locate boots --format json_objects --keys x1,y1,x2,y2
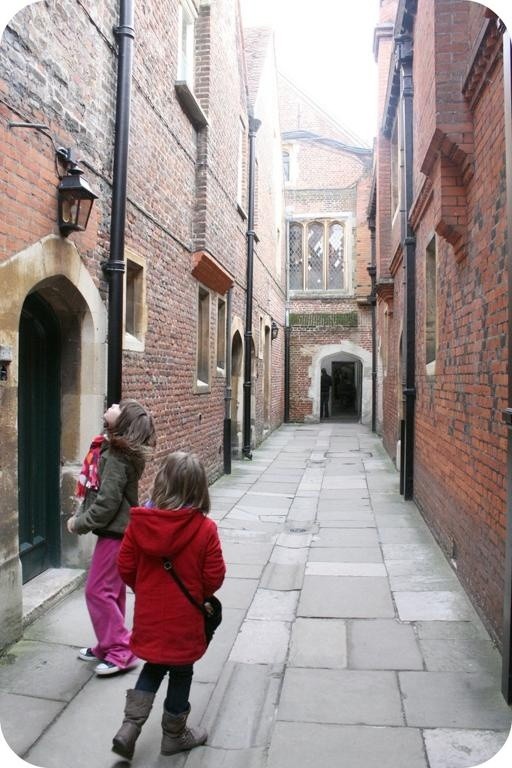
[
  {"x1": 160, "y1": 699, "x2": 208, "y2": 756},
  {"x1": 111, "y1": 688, "x2": 155, "y2": 761}
]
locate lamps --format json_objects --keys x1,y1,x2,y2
[{"x1": 8, "y1": 122, "x2": 98, "y2": 237}]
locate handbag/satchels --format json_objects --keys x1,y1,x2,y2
[{"x1": 203, "y1": 594, "x2": 222, "y2": 647}]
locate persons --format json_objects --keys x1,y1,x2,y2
[
  {"x1": 106, "y1": 446, "x2": 227, "y2": 759},
  {"x1": 67, "y1": 396, "x2": 160, "y2": 679},
  {"x1": 320, "y1": 368, "x2": 333, "y2": 420}
]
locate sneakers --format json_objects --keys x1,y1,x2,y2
[
  {"x1": 79, "y1": 646, "x2": 101, "y2": 662},
  {"x1": 94, "y1": 654, "x2": 140, "y2": 675}
]
